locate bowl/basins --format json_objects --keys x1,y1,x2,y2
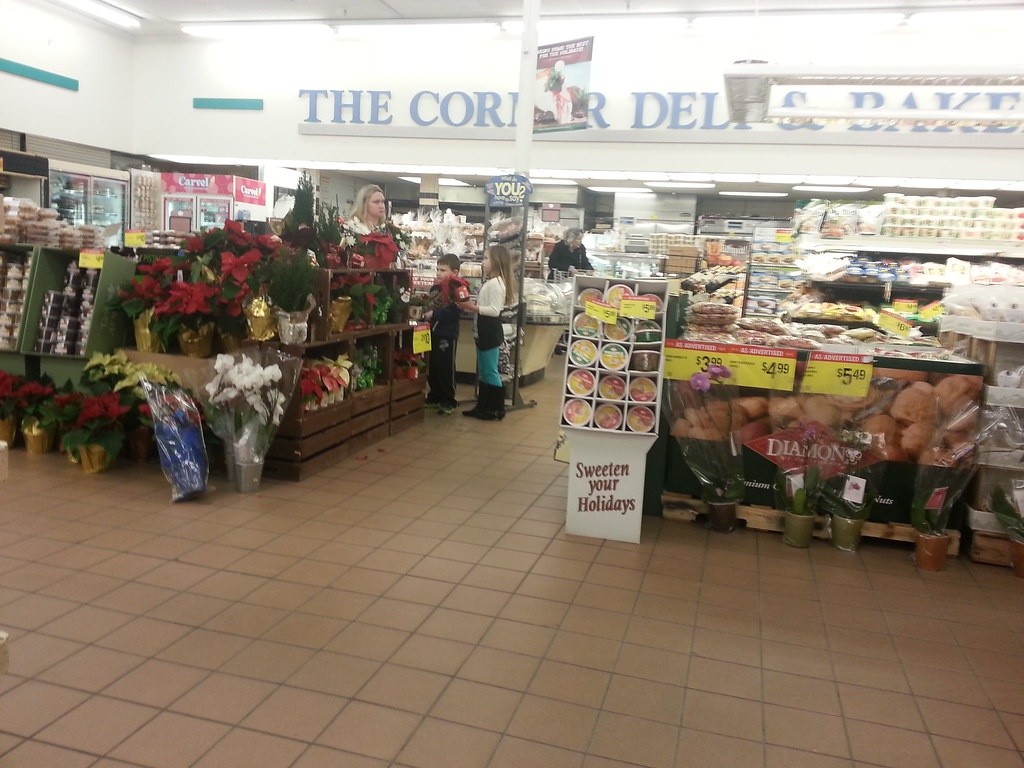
[{"x1": 882, "y1": 192, "x2": 1024, "y2": 241}]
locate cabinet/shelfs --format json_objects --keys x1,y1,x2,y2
[
  {"x1": 788, "y1": 233, "x2": 1024, "y2": 337},
  {"x1": 741, "y1": 252, "x2": 800, "y2": 317}
]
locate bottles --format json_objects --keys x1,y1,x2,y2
[{"x1": 680, "y1": 264, "x2": 747, "y2": 305}]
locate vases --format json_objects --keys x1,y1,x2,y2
[
  {"x1": 0, "y1": 416, "x2": 17, "y2": 449},
  {"x1": 235, "y1": 461, "x2": 264, "y2": 492},
  {"x1": 78, "y1": 444, "x2": 112, "y2": 473},
  {"x1": 274, "y1": 294, "x2": 316, "y2": 344},
  {"x1": 331, "y1": 297, "x2": 352, "y2": 332},
  {"x1": 832, "y1": 514, "x2": 863, "y2": 551},
  {"x1": 405, "y1": 367, "x2": 418, "y2": 378},
  {"x1": 244, "y1": 295, "x2": 277, "y2": 342},
  {"x1": 306, "y1": 385, "x2": 343, "y2": 411},
  {"x1": 782, "y1": 508, "x2": 817, "y2": 547},
  {"x1": 22, "y1": 421, "x2": 55, "y2": 453},
  {"x1": 133, "y1": 307, "x2": 166, "y2": 353},
  {"x1": 127, "y1": 428, "x2": 151, "y2": 456},
  {"x1": 707, "y1": 501, "x2": 736, "y2": 533},
  {"x1": 177, "y1": 321, "x2": 215, "y2": 358}
]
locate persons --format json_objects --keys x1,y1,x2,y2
[
  {"x1": 422, "y1": 254, "x2": 461, "y2": 416},
  {"x1": 349, "y1": 184, "x2": 385, "y2": 227},
  {"x1": 547, "y1": 227, "x2": 594, "y2": 355},
  {"x1": 455, "y1": 245, "x2": 516, "y2": 421}
]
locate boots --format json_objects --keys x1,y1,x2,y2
[
  {"x1": 462, "y1": 382, "x2": 486, "y2": 416},
  {"x1": 476, "y1": 384, "x2": 507, "y2": 419}
]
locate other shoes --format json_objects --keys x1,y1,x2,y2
[
  {"x1": 437, "y1": 402, "x2": 457, "y2": 415},
  {"x1": 424, "y1": 399, "x2": 440, "y2": 408}
]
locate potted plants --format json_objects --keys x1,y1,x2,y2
[
  {"x1": 992, "y1": 485, "x2": 1024, "y2": 577},
  {"x1": 910, "y1": 467, "x2": 959, "y2": 571}
]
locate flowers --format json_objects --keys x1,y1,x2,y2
[
  {"x1": 781, "y1": 420, "x2": 877, "y2": 520},
  {"x1": 0, "y1": 350, "x2": 204, "y2": 473},
  {"x1": 301, "y1": 200, "x2": 426, "y2": 406},
  {"x1": 689, "y1": 364, "x2": 745, "y2": 503},
  {"x1": 206, "y1": 353, "x2": 285, "y2": 460},
  {"x1": 108, "y1": 220, "x2": 298, "y2": 354}
]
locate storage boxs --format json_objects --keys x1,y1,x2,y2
[{"x1": 663, "y1": 243, "x2": 699, "y2": 275}]
